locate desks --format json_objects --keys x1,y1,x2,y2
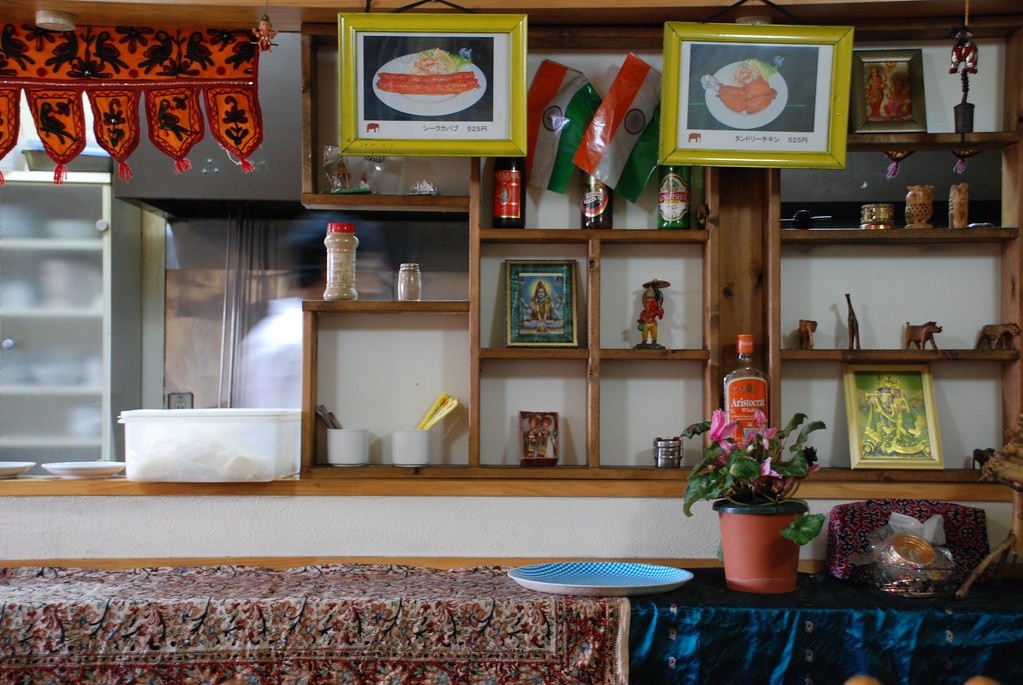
[{"x1": 1, "y1": 567, "x2": 1023, "y2": 685}]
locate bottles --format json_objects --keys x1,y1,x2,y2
[
  {"x1": 398, "y1": 263, "x2": 421, "y2": 301},
  {"x1": 581, "y1": 171, "x2": 612, "y2": 230},
  {"x1": 721, "y1": 333, "x2": 768, "y2": 443},
  {"x1": 323, "y1": 223, "x2": 360, "y2": 300},
  {"x1": 492, "y1": 156, "x2": 526, "y2": 229},
  {"x1": 658, "y1": 164, "x2": 691, "y2": 230}
]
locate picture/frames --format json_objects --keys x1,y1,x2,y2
[
  {"x1": 504, "y1": 259, "x2": 576, "y2": 347},
  {"x1": 519, "y1": 410, "x2": 560, "y2": 463},
  {"x1": 843, "y1": 362, "x2": 944, "y2": 469},
  {"x1": 656, "y1": 21, "x2": 855, "y2": 170},
  {"x1": 852, "y1": 48, "x2": 928, "y2": 132},
  {"x1": 337, "y1": 12, "x2": 528, "y2": 158}
]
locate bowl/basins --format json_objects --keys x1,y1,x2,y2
[{"x1": 873, "y1": 562, "x2": 954, "y2": 597}]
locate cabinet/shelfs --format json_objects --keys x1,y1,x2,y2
[
  {"x1": 0, "y1": 170, "x2": 141, "y2": 466},
  {"x1": 301, "y1": 0, "x2": 1023, "y2": 481}
]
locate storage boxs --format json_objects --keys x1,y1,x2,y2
[{"x1": 120, "y1": 407, "x2": 301, "y2": 482}]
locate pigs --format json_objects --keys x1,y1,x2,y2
[{"x1": 905, "y1": 322, "x2": 942, "y2": 351}]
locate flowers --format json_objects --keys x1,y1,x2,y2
[{"x1": 681, "y1": 400, "x2": 827, "y2": 514}]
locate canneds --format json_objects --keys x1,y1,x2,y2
[
  {"x1": 324, "y1": 223, "x2": 359, "y2": 300},
  {"x1": 396, "y1": 264, "x2": 422, "y2": 302}
]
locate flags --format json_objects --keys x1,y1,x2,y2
[
  {"x1": 525, "y1": 59, "x2": 604, "y2": 193},
  {"x1": 571, "y1": 52, "x2": 665, "y2": 204}
]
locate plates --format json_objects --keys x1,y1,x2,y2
[
  {"x1": 507, "y1": 562, "x2": 694, "y2": 598},
  {"x1": 372, "y1": 53, "x2": 486, "y2": 116},
  {"x1": 41, "y1": 461, "x2": 125, "y2": 480},
  {"x1": 0, "y1": 461, "x2": 36, "y2": 479},
  {"x1": 705, "y1": 61, "x2": 788, "y2": 129}
]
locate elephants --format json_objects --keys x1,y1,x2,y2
[{"x1": 798, "y1": 319, "x2": 817, "y2": 351}]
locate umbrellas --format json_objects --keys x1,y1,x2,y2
[{"x1": 642, "y1": 279, "x2": 670, "y2": 308}]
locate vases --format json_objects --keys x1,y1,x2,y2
[{"x1": 714, "y1": 503, "x2": 810, "y2": 593}]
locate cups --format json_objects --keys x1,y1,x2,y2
[
  {"x1": 326, "y1": 426, "x2": 369, "y2": 467},
  {"x1": 391, "y1": 428, "x2": 432, "y2": 468}
]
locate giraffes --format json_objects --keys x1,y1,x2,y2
[{"x1": 845, "y1": 294, "x2": 861, "y2": 349}]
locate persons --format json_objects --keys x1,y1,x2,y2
[
  {"x1": 252, "y1": 20, "x2": 277, "y2": 53},
  {"x1": 522, "y1": 416, "x2": 552, "y2": 458},
  {"x1": 637, "y1": 286, "x2": 664, "y2": 344},
  {"x1": 243, "y1": 215, "x2": 329, "y2": 410}
]
